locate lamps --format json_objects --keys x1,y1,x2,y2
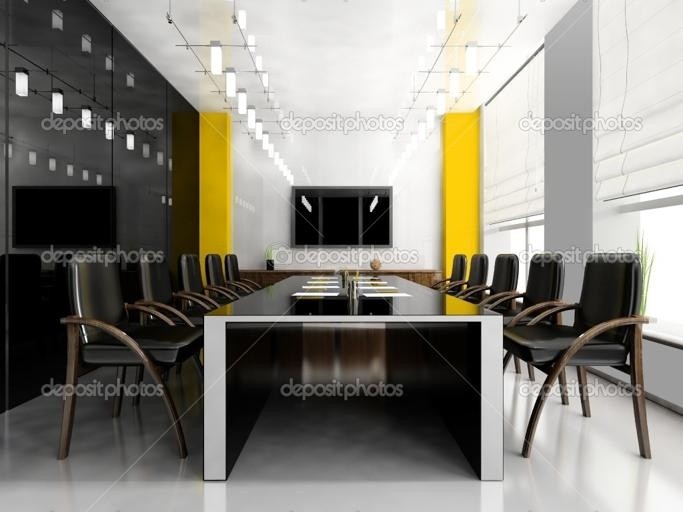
[
  {"x1": 1, "y1": 0, "x2": 173, "y2": 207},
  {"x1": 230, "y1": 2, "x2": 270, "y2": 89},
  {"x1": 164, "y1": 0, "x2": 295, "y2": 187},
  {"x1": 409, "y1": 6, "x2": 476, "y2": 150}
]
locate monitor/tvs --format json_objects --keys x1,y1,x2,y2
[
  {"x1": 12, "y1": 186, "x2": 117, "y2": 247},
  {"x1": 291, "y1": 185, "x2": 393, "y2": 247}
]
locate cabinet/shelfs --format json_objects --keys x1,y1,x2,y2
[{"x1": 240, "y1": 268, "x2": 443, "y2": 289}]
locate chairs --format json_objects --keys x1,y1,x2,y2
[
  {"x1": 429, "y1": 253, "x2": 653, "y2": 460},
  {"x1": 57, "y1": 254, "x2": 263, "y2": 460}
]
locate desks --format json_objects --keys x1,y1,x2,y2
[{"x1": 203, "y1": 274, "x2": 504, "y2": 482}]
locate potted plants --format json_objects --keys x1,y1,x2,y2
[{"x1": 265, "y1": 246, "x2": 276, "y2": 270}]
